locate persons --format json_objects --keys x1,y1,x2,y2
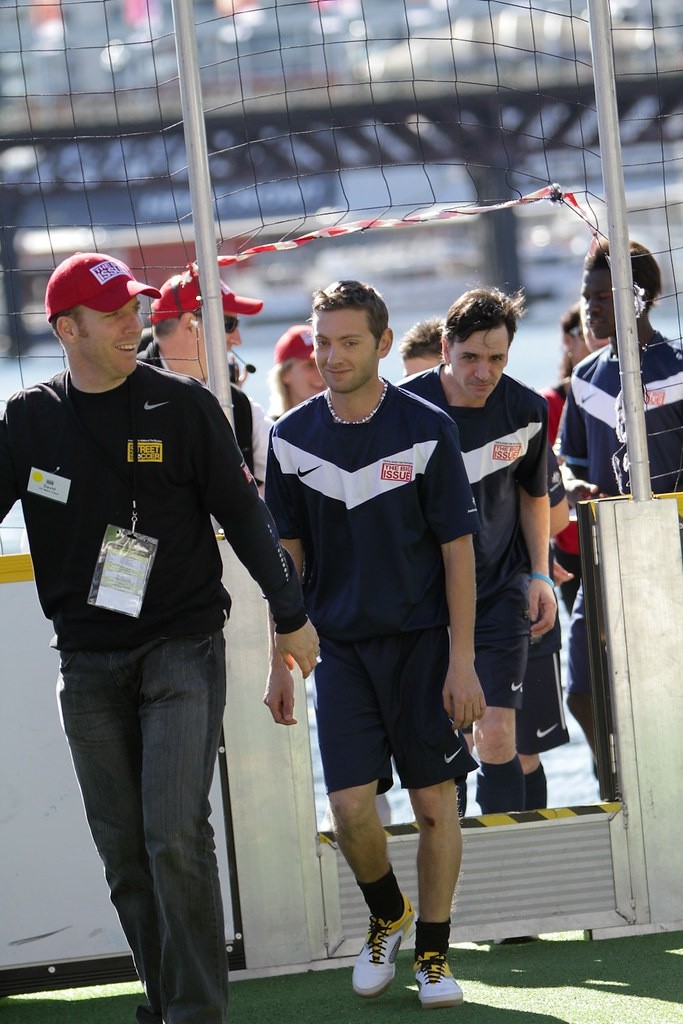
[
  {"x1": 140, "y1": 271, "x2": 274, "y2": 507},
  {"x1": 541, "y1": 240, "x2": 683, "y2": 797},
  {"x1": 393, "y1": 286, "x2": 569, "y2": 817},
  {"x1": 270, "y1": 325, "x2": 329, "y2": 422},
  {"x1": 263, "y1": 281, "x2": 485, "y2": 1007},
  {"x1": 0, "y1": 251, "x2": 319, "y2": 1024}
]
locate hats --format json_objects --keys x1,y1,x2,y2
[
  {"x1": 147, "y1": 272, "x2": 264, "y2": 324},
  {"x1": 274, "y1": 325, "x2": 314, "y2": 363},
  {"x1": 45, "y1": 252, "x2": 164, "y2": 323}
]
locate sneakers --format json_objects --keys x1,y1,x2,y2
[
  {"x1": 413, "y1": 950, "x2": 464, "y2": 1009},
  {"x1": 352, "y1": 891, "x2": 418, "y2": 998}
]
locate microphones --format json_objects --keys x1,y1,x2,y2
[{"x1": 230, "y1": 350, "x2": 256, "y2": 373}]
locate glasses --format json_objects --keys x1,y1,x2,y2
[
  {"x1": 569, "y1": 327, "x2": 583, "y2": 338},
  {"x1": 224, "y1": 316, "x2": 239, "y2": 332}
]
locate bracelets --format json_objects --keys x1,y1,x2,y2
[{"x1": 532, "y1": 574, "x2": 555, "y2": 588}]
